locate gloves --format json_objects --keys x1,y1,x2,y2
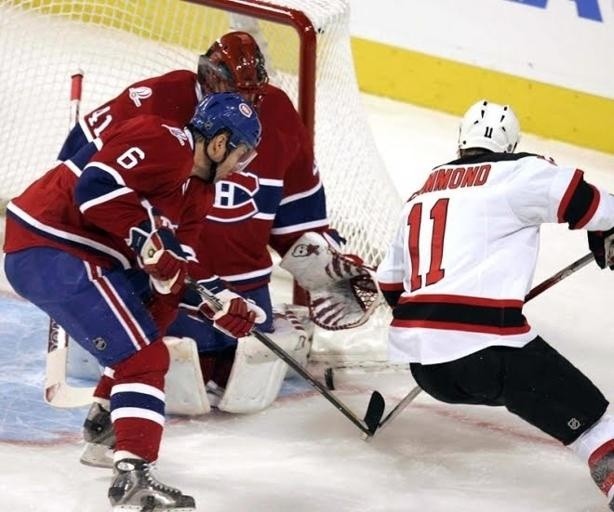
[
  {"x1": 279, "y1": 231, "x2": 383, "y2": 330},
  {"x1": 136, "y1": 227, "x2": 188, "y2": 297},
  {"x1": 587, "y1": 228, "x2": 614, "y2": 272},
  {"x1": 198, "y1": 287, "x2": 267, "y2": 338}
]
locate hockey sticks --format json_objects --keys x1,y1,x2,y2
[
  {"x1": 44, "y1": 66, "x2": 98, "y2": 407},
  {"x1": 192, "y1": 279, "x2": 385, "y2": 436}
]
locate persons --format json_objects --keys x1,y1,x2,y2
[
  {"x1": 376, "y1": 100, "x2": 614, "y2": 512},
  {"x1": 53, "y1": 32, "x2": 349, "y2": 416},
  {"x1": 3, "y1": 94, "x2": 266, "y2": 512}
]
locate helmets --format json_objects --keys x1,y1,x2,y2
[
  {"x1": 456, "y1": 99, "x2": 522, "y2": 156},
  {"x1": 189, "y1": 30, "x2": 269, "y2": 175}
]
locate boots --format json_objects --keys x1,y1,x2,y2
[
  {"x1": 79, "y1": 401, "x2": 117, "y2": 447},
  {"x1": 107, "y1": 457, "x2": 195, "y2": 509}
]
